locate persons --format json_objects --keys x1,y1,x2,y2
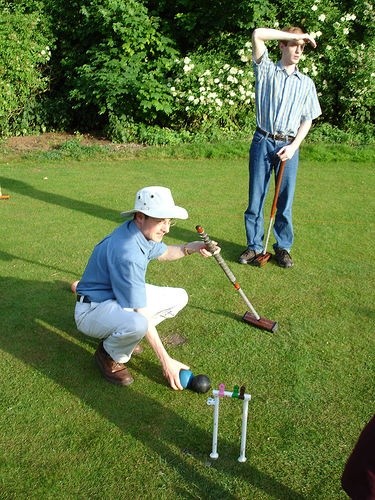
[
  {"x1": 74, "y1": 186, "x2": 222, "y2": 390},
  {"x1": 238, "y1": 27, "x2": 322, "y2": 268}
]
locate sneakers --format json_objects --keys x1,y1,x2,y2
[
  {"x1": 238, "y1": 248, "x2": 256, "y2": 264},
  {"x1": 275, "y1": 250, "x2": 295, "y2": 266}
]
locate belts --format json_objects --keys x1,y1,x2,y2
[
  {"x1": 76, "y1": 294, "x2": 91, "y2": 304},
  {"x1": 256, "y1": 127, "x2": 295, "y2": 142}
]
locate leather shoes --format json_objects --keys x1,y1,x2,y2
[
  {"x1": 94, "y1": 342, "x2": 134, "y2": 385},
  {"x1": 133, "y1": 344, "x2": 143, "y2": 353}
]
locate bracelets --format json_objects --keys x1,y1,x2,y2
[{"x1": 184, "y1": 247, "x2": 191, "y2": 256}]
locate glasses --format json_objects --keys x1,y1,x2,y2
[{"x1": 148, "y1": 216, "x2": 177, "y2": 226}]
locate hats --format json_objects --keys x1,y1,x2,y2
[{"x1": 120, "y1": 186, "x2": 188, "y2": 220}]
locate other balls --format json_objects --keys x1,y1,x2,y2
[{"x1": 179, "y1": 369, "x2": 211, "y2": 394}]
